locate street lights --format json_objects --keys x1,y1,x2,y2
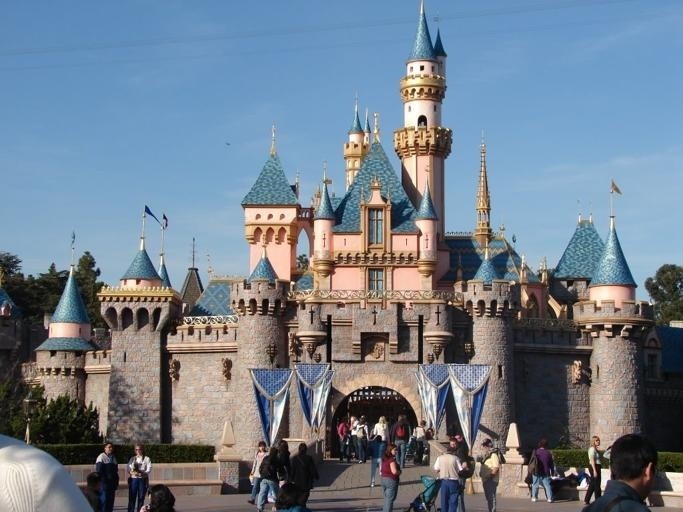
[{"x1": 21, "y1": 391, "x2": 37, "y2": 443}]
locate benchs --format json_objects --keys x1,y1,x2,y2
[
  {"x1": 515, "y1": 482, "x2": 682, "y2": 507},
  {"x1": 75, "y1": 478, "x2": 223, "y2": 499}
]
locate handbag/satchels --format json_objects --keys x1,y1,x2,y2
[
  {"x1": 248, "y1": 473, "x2": 255, "y2": 485},
  {"x1": 527, "y1": 456, "x2": 539, "y2": 475},
  {"x1": 523, "y1": 476, "x2": 533, "y2": 484}
]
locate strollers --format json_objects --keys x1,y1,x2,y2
[{"x1": 404, "y1": 473, "x2": 443, "y2": 512}]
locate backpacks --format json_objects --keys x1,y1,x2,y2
[{"x1": 396, "y1": 422, "x2": 407, "y2": 438}]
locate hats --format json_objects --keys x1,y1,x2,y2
[{"x1": 480, "y1": 438, "x2": 492, "y2": 445}]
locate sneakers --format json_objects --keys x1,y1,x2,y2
[
  {"x1": 338, "y1": 455, "x2": 367, "y2": 466},
  {"x1": 530, "y1": 497, "x2": 538, "y2": 503},
  {"x1": 247, "y1": 498, "x2": 269, "y2": 512},
  {"x1": 368, "y1": 481, "x2": 376, "y2": 488},
  {"x1": 547, "y1": 498, "x2": 553, "y2": 503},
  {"x1": 583, "y1": 499, "x2": 590, "y2": 505}
]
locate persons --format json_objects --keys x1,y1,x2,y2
[
  {"x1": 337, "y1": 409, "x2": 505, "y2": 512},
  {"x1": 138, "y1": 483, "x2": 177, "y2": 512},
  {"x1": 97, "y1": 444, "x2": 119, "y2": 512},
  {"x1": 584, "y1": 435, "x2": 608, "y2": 503},
  {"x1": 579, "y1": 436, "x2": 662, "y2": 512},
  {"x1": 0, "y1": 431, "x2": 95, "y2": 512},
  {"x1": 128, "y1": 444, "x2": 154, "y2": 512},
  {"x1": 85, "y1": 472, "x2": 106, "y2": 512},
  {"x1": 251, "y1": 441, "x2": 320, "y2": 512},
  {"x1": 528, "y1": 439, "x2": 555, "y2": 503}
]
